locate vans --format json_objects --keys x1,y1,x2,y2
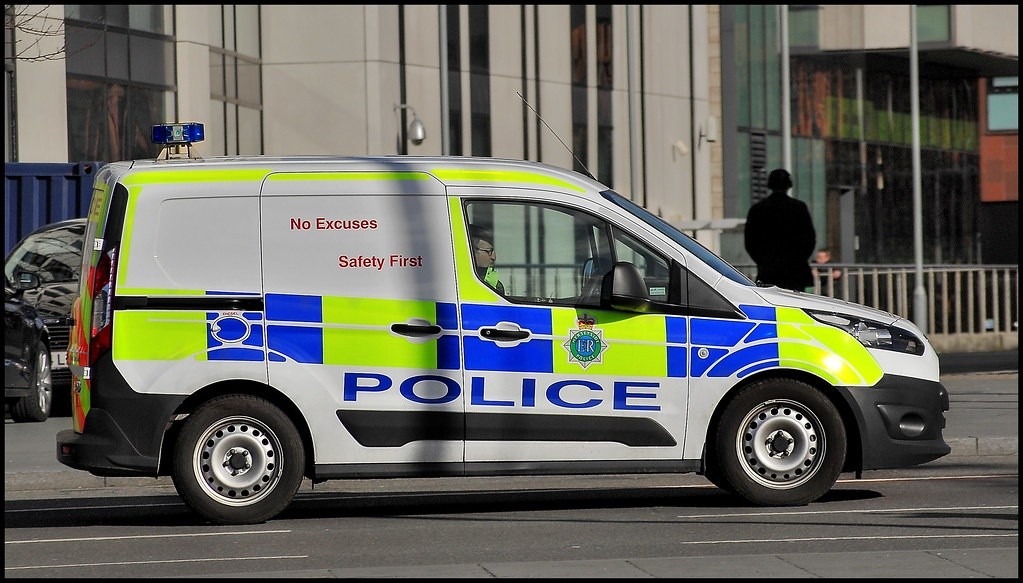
[{"x1": 55, "y1": 123, "x2": 951, "y2": 523}]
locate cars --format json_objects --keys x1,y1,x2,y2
[
  {"x1": 4, "y1": 218, "x2": 88, "y2": 416},
  {"x1": 4, "y1": 272, "x2": 52, "y2": 422}
]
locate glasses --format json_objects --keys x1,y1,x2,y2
[{"x1": 474, "y1": 247, "x2": 493, "y2": 256}]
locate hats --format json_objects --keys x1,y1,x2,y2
[{"x1": 767, "y1": 169, "x2": 793, "y2": 190}]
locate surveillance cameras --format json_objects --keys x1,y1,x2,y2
[{"x1": 409, "y1": 118, "x2": 426, "y2": 145}]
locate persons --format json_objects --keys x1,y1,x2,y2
[
  {"x1": 812, "y1": 248, "x2": 842, "y2": 295},
  {"x1": 469, "y1": 224, "x2": 503, "y2": 296},
  {"x1": 744, "y1": 169, "x2": 815, "y2": 292}
]
